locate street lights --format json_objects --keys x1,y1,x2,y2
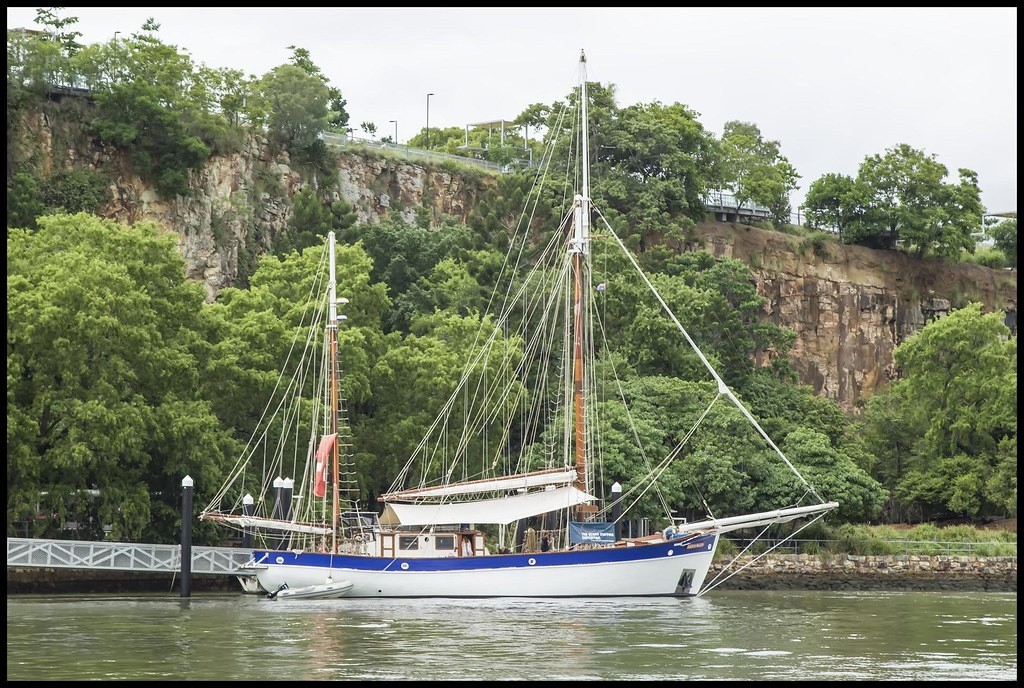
[
  {"x1": 114, "y1": 31, "x2": 121, "y2": 77},
  {"x1": 389, "y1": 120, "x2": 398, "y2": 145},
  {"x1": 427, "y1": 94, "x2": 434, "y2": 150}
]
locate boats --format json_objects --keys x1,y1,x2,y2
[{"x1": 267, "y1": 578, "x2": 354, "y2": 599}]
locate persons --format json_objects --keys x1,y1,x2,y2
[
  {"x1": 460, "y1": 536, "x2": 473, "y2": 558},
  {"x1": 541, "y1": 532, "x2": 550, "y2": 552}
]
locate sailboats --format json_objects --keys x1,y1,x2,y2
[{"x1": 196, "y1": 47, "x2": 841, "y2": 598}]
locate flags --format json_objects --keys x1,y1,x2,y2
[
  {"x1": 597, "y1": 283, "x2": 605, "y2": 290},
  {"x1": 313, "y1": 434, "x2": 338, "y2": 497}
]
